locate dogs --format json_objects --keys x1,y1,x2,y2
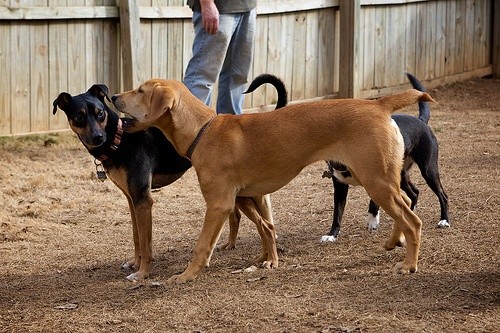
[
  {"x1": 52, "y1": 74, "x2": 288, "y2": 282},
  {"x1": 110, "y1": 79, "x2": 438, "y2": 288},
  {"x1": 318, "y1": 72, "x2": 452, "y2": 243}
]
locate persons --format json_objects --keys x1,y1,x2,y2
[{"x1": 186, "y1": 0, "x2": 258, "y2": 115}]
